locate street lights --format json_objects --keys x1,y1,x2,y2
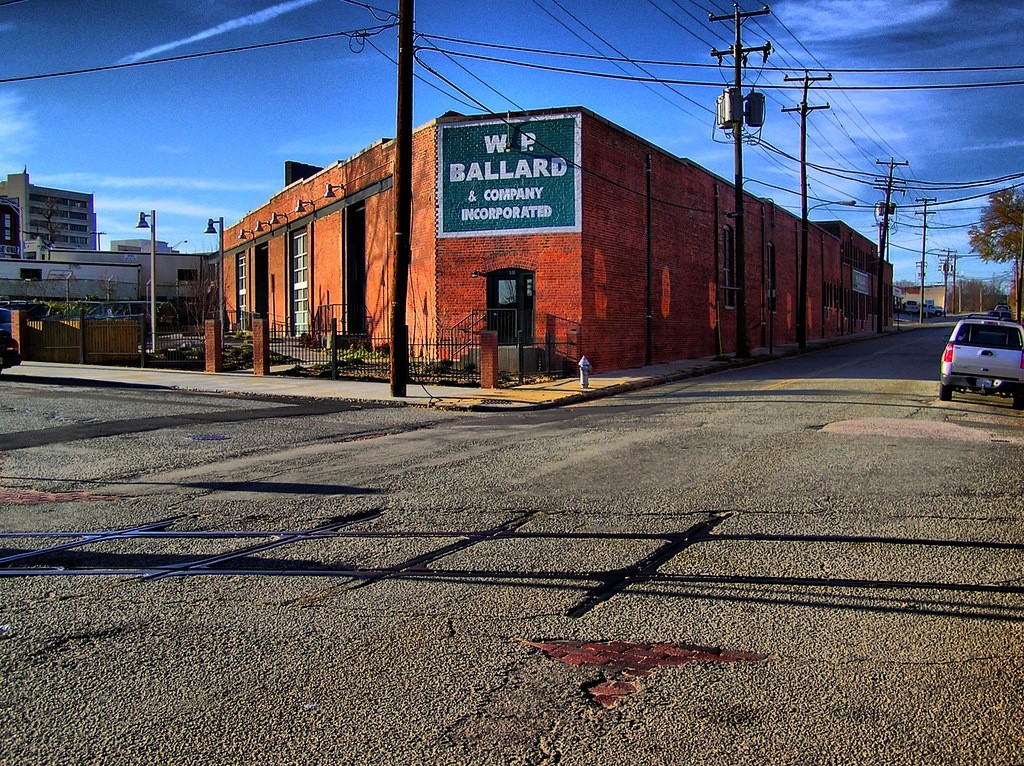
[
  {"x1": 799, "y1": 200, "x2": 856, "y2": 347},
  {"x1": 919, "y1": 249, "x2": 946, "y2": 324},
  {"x1": 135, "y1": 209, "x2": 156, "y2": 359},
  {"x1": 204, "y1": 217, "x2": 225, "y2": 349}
]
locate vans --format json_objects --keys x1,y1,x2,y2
[
  {"x1": 989, "y1": 310, "x2": 1013, "y2": 319},
  {"x1": 904, "y1": 301, "x2": 919, "y2": 312}
]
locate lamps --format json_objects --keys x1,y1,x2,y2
[
  {"x1": 237, "y1": 229, "x2": 253, "y2": 239},
  {"x1": 323, "y1": 183, "x2": 345, "y2": 198},
  {"x1": 270, "y1": 212, "x2": 288, "y2": 224},
  {"x1": 295, "y1": 199, "x2": 315, "y2": 212},
  {"x1": 255, "y1": 220, "x2": 272, "y2": 231}
]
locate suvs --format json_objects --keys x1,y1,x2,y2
[
  {"x1": 922, "y1": 305, "x2": 943, "y2": 317},
  {"x1": 939, "y1": 313, "x2": 1024, "y2": 409}
]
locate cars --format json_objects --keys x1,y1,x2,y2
[
  {"x1": 994, "y1": 305, "x2": 1011, "y2": 311},
  {"x1": 0, "y1": 329, "x2": 23, "y2": 374},
  {"x1": 0, "y1": 299, "x2": 181, "y2": 344}
]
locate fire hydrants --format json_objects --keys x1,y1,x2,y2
[{"x1": 578, "y1": 356, "x2": 591, "y2": 388}]
